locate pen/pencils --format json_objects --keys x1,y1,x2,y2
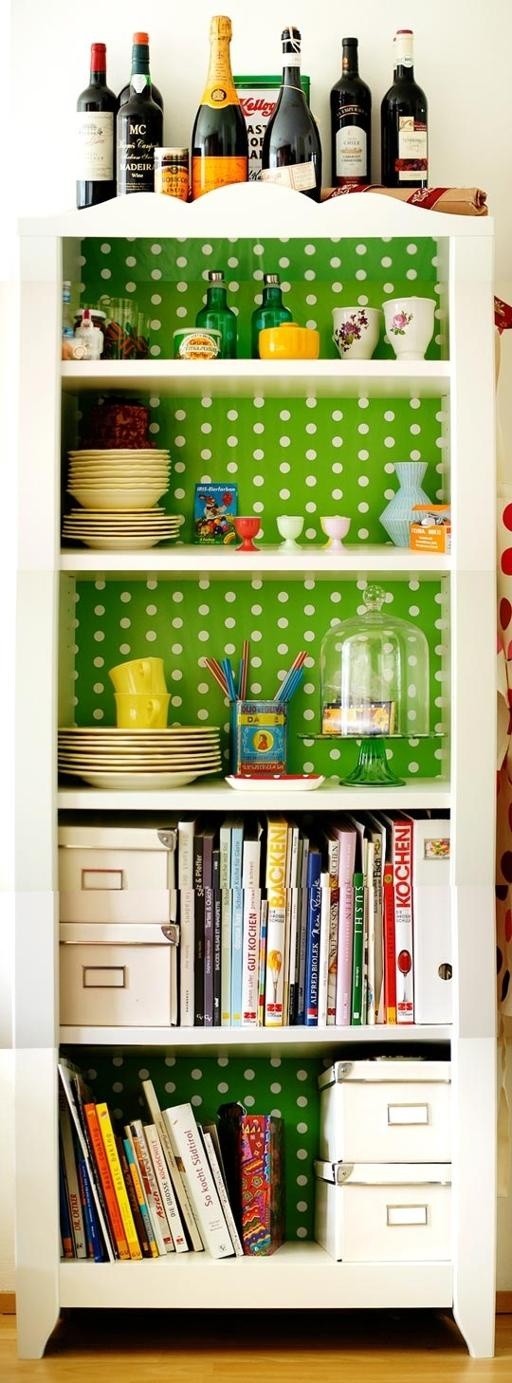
[{"x1": 204, "y1": 640, "x2": 309, "y2": 702}]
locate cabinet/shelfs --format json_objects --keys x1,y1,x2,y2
[{"x1": 12, "y1": 179, "x2": 497, "y2": 1362}]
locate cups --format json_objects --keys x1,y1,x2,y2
[
  {"x1": 108, "y1": 655, "x2": 167, "y2": 694},
  {"x1": 230, "y1": 695, "x2": 291, "y2": 774},
  {"x1": 329, "y1": 305, "x2": 382, "y2": 358},
  {"x1": 380, "y1": 296, "x2": 436, "y2": 361},
  {"x1": 112, "y1": 690, "x2": 172, "y2": 729},
  {"x1": 75, "y1": 293, "x2": 153, "y2": 359}
]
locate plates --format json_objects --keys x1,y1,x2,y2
[
  {"x1": 62, "y1": 444, "x2": 183, "y2": 551},
  {"x1": 223, "y1": 771, "x2": 327, "y2": 790},
  {"x1": 57, "y1": 724, "x2": 223, "y2": 788}
]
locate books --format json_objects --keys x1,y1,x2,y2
[
  {"x1": 56, "y1": 1057, "x2": 287, "y2": 1260},
  {"x1": 176, "y1": 813, "x2": 416, "y2": 1026}
]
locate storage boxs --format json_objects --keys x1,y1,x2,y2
[
  {"x1": 312, "y1": 1057, "x2": 456, "y2": 1264},
  {"x1": 60, "y1": 819, "x2": 181, "y2": 1027}
]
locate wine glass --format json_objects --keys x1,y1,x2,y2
[
  {"x1": 319, "y1": 514, "x2": 351, "y2": 550},
  {"x1": 275, "y1": 514, "x2": 305, "y2": 552},
  {"x1": 231, "y1": 515, "x2": 262, "y2": 553}
]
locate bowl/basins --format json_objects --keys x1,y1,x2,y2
[{"x1": 255, "y1": 324, "x2": 322, "y2": 359}]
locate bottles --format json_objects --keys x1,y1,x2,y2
[
  {"x1": 261, "y1": 22, "x2": 322, "y2": 203},
  {"x1": 329, "y1": 31, "x2": 373, "y2": 188},
  {"x1": 377, "y1": 27, "x2": 431, "y2": 188},
  {"x1": 250, "y1": 271, "x2": 294, "y2": 358},
  {"x1": 116, "y1": 30, "x2": 163, "y2": 195},
  {"x1": 62, "y1": 280, "x2": 74, "y2": 338},
  {"x1": 196, "y1": 269, "x2": 238, "y2": 357},
  {"x1": 71, "y1": 42, "x2": 116, "y2": 209},
  {"x1": 188, "y1": 11, "x2": 250, "y2": 202}
]
